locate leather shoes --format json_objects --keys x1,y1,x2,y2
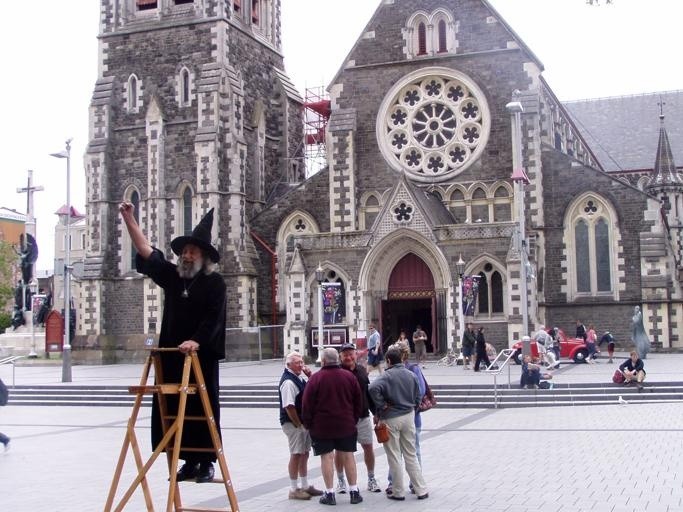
[
  {"x1": 168, "y1": 464, "x2": 200, "y2": 480},
  {"x1": 196, "y1": 464, "x2": 214, "y2": 483}
]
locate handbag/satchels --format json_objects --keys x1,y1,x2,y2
[
  {"x1": 409, "y1": 364, "x2": 436, "y2": 410},
  {"x1": 375, "y1": 424, "x2": 388, "y2": 443}
]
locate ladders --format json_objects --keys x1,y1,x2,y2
[{"x1": 104, "y1": 345, "x2": 241, "y2": 512}]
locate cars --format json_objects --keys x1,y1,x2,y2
[{"x1": 511, "y1": 324, "x2": 601, "y2": 366}]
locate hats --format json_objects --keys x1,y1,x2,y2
[
  {"x1": 171, "y1": 208, "x2": 220, "y2": 263},
  {"x1": 339, "y1": 343, "x2": 355, "y2": 351}
]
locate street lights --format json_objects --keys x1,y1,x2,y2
[
  {"x1": 312, "y1": 256, "x2": 325, "y2": 367},
  {"x1": 50, "y1": 135, "x2": 78, "y2": 383},
  {"x1": 454, "y1": 252, "x2": 484, "y2": 367},
  {"x1": 503, "y1": 96, "x2": 536, "y2": 371}
]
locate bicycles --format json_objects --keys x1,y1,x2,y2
[{"x1": 436, "y1": 349, "x2": 455, "y2": 367}]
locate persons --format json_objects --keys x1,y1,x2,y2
[
  {"x1": 533, "y1": 325, "x2": 560, "y2": 370},
  {"x1": 598, "y1": 331, "x2": 616, "y2": 364},
  {"x1": 119, "y1": 200, "x2": 228, "y2": 484},
  {"x1": 519, "y1": 355, "x2": 541, "y2": 390},
  {"x1": 398, "y1": 332, "x2": 409, "y2": 347},
  {"x1": 584, "y1": 324, "x2": 596, "y2": 364},
  {"x1": 461, "y1": 323, "x2": 477, "y2": 370},
  {"x1": 366, "y1": 324, "x2": 385, "y2": 376},
  {"x1": 618, "y1": 350, "x2": 646, "y2": 384},
  {"x1": 278, "y1": 342, "x2": 429, "y2": 506},
  {"x1": 575, "y1": 319, "x2": 587, "y2": 340},
  {"x1": 412, "y1": 326, "x2": 428, "y2": 368},
  {"x1": 473, "y1": 326, "x2": 494, "y2": 372}
]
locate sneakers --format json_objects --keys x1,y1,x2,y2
[
  {"x1": 288, "y1": 480, "x2": 336, "y2": 505},
  {"x1": 368, "y1": 478, "x2": 381, "y2": 491},
  {"x1": 385, "y1": 481, "x2": 428, "y2": 499},
  {"x1": 350, "y1": 490, "x2": 362, "y2": 504},
  {"x1": 337, "y1": 479, "x2": 345, "y2": 493}
]
